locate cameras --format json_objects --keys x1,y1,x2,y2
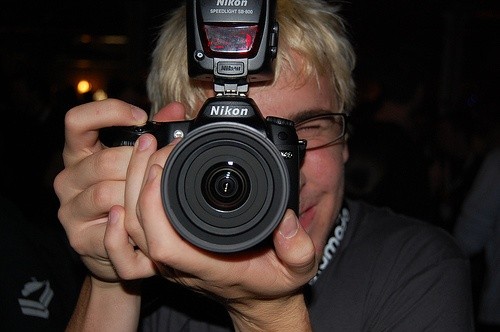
[{"x1": 98, "y1": 0, "x2": 307, "y2": 255}]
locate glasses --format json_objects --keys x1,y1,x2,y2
[{"x1": 295, "y1": 112, "x2": 348, "y2": 150}]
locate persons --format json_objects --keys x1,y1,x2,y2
[
  {"x1": 448, "y1": 146, "x2": 500, "y2": 332},
  {"x1": 53, "y1": 0, "x2": 472, "y2": 332}
]
